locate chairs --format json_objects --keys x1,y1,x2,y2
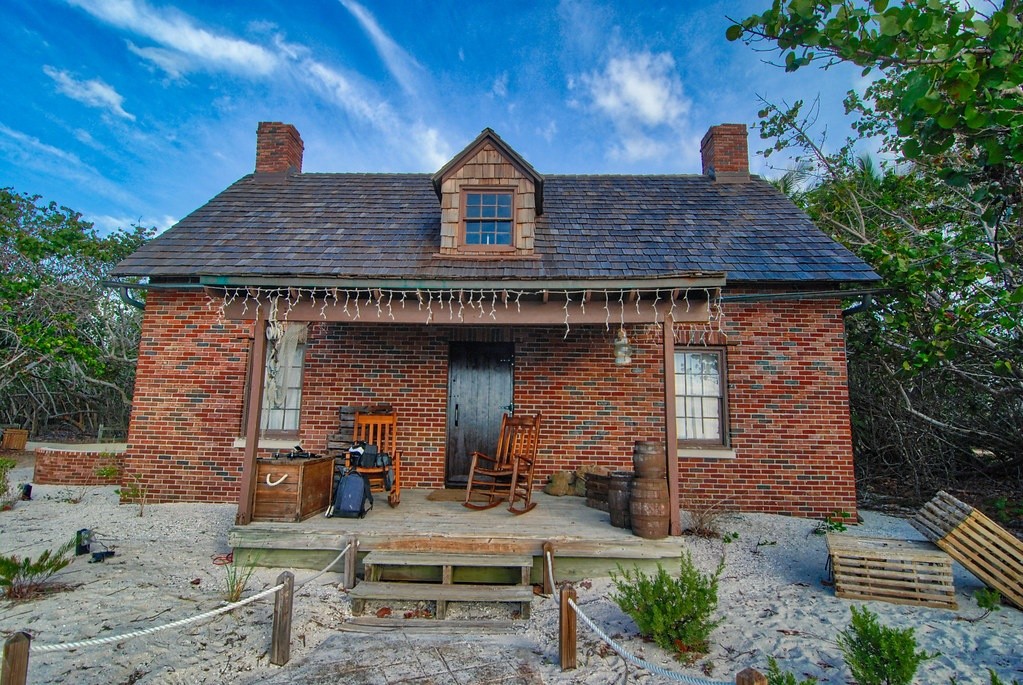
[
  {"x1": 462, "y1": 412, "x2": 542, "y2": 515},
  {"x1": 345, "y1": 408, "x2": 401, "y2": 509}
]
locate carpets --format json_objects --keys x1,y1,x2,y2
[{"x1": 426, "y1": 489, "x2": 521, "y2": 503}]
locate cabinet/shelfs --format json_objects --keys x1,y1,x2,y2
[{"x1": 251, "y1": 455, "x2": 337, "y2": 522}]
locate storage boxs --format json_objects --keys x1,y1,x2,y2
[
  {"x1": 824, "y1": 532, "x2": 959, "y2": 611},
  {"x1": 326, "y1": 405, "x2": 393, "y2": 493},
  {"x1": 908, "y1": 489, "x2": 1023, "y2": 610}
]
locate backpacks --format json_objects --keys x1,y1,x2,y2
[{"x1": 324, "y1": 469, "x2": 374, "y2": 519}]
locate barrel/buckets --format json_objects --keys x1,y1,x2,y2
[
  {"x1": 609, "y1": 474, "x2": 637, "y2": 527},
  {"x1": 633, "y1": 441, "x2": 666, "y2": 479},
  {"x1": 630, "y1": 478, "x2": 670, "y2": 540}
]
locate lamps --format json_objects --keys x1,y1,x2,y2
[{"x1": 614, "y1": 323, "x2": 632, "y2": 367}]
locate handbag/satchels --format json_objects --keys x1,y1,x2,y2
[{"x1": 375, "y1": 450, "x2": 389, "y2": 467}]
